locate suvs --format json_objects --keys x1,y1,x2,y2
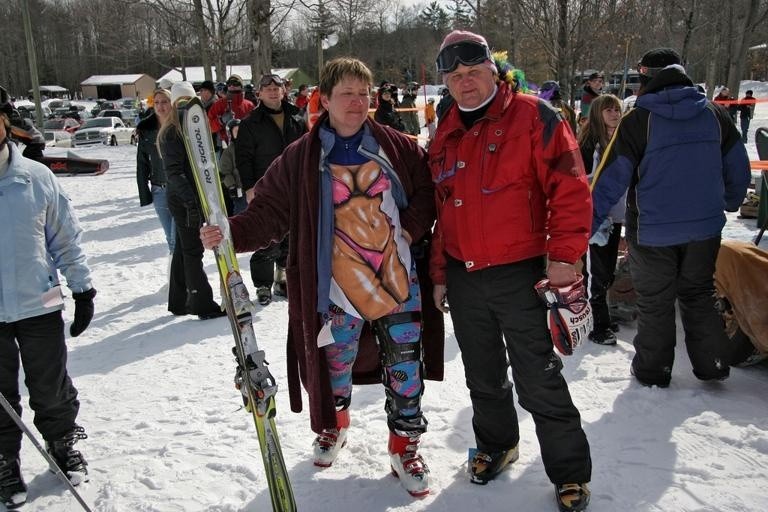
[{"x1": 609, "y1": 71, "x2": 642, "y2": 97}]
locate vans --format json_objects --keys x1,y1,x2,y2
[{"x1": 574, "y1": 70, "x2": 605, "y2": 100}]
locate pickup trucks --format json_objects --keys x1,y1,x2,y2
[
  {"x1": 92, "y1": 101, "x2": 120, "y2": 116},
  {"x1": 70, "y1": 117, "x2": 138, "y2": 148},
  {"x1": 96, "y1": 110, "x2": 136, "y2": 127}
]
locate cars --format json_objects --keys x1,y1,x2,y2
[
  {"x1": 44, "y1": 131, "x2": 72, "y2": 147},
  {"x1": 44, "y1": 118, "x2": 80, "y2": 133},
  {"x1": 42, "y1": 99, "x2": 85, "y2": 115}
]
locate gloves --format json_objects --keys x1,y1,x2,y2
[
  {"x1": 589, "y1": 217, "x2": 612, "y2": 246},
  {"x1": 217, "y1": 110, "x2": 234, "y2": 126},
  {"x1": 549, "y1": 271, "x2": 594, "y2": 351},
  {"x1": 228, "y1": 185, "x2": 239, "y2": 201},
  {"x1": 536, "y1": 280, "x2": 572, "y2": 357},
  {"x1": 70, "y1": 288, "x2": 97, "y2": 338},
  {"x1": 184, "y1": 199, "x2": 200, "y2": 232}
]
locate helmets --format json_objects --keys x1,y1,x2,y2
[
  {"x1": 428, "y1": 98, "x2": 434, "y2": 103},
  {"x1": 438, "y1": 87, "x2": 448, "y2": 96},
  {"x1": 245, "y1": 83, "x2": 256, "y2": 91},
  {"x1": 0, "y1": 86, "x2": 14, "y2": 117},
  {"x1": 402, "y1": 82, "x2": 420, "y2": 95}
]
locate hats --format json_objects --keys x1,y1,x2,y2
[
  {"x1": 160, "y1": 76, "x2": 174, "y2": 89},
  {"x1": 171, "y1": 82, "x2": 198, "y2": 106},
  {"x1": 380, "y1": 80, "x2": 389, "y2": 87},
  {"x1": 539, "y1": 81, "x2": 560, "y2": 92},
  {"x1": 439, "y1": 30, "x2": 497, "y2": 81},
  {"x1": 199, "y1": 81, "x2": 215, "y2": 94},
  {"x1": 589, "y1": 72, "x2": 604, "y2": 81},
  {"x1": 227, "y1": 74, "x2": 244, "y2": 89},
  {"x1": 373, "y1": 87, "x2": 393, "y2": 98}
]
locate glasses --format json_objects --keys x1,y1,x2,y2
[
  {"x1": 216, "y1": 86, "x2": 225, "y2": 91},
  {"x1": 435, "y1": 41, "x2": 494, "y2": 73},
  {"x1": 591, "y1": 80, "x2": 602, "y2": 83},
  {"x1": 226, "y1": 80, "x2": 241, "y2": 87},
  {"x1": 259, "y1": 74, "x2": 283, "y2": 89}
]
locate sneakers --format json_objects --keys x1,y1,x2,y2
[
  {"x1": 256, "y1": 284, "x2": 272, "y2": 306},
  {"x1": 387, "y1": 411, "x2": 435, "y2": 498},
  {"x1": 469, "y1": 443, "x2": 518, "y2": 485},
  {"x1": 630, "y1": 362, "x2": 672, "y2": 388},
  {"x1": 45, "y1": 425, "x2": 89, "y2": 486},
  {"x1": 273, "y1": 264, "x2": 287, "y2": 300},
  {"x1": 0, "y1": 455, "x2": 28, "y2": 509},
  {"x1": 589, "y1": 330, "x2": 616, "y2": 345},
  {"x1": 312, "y1": 394, "x2": 351, "y2": 468},
  {"x1": 692, "y1": 366, "x2": 730, "y2": 382},
  {"x1": 555, "y1": 483, "x2": 591, "y2": 512},
  {"x1": 198, "y1": 301, "x2": 227, "y2": 321}
]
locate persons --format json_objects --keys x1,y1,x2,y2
[
  {"x1": 427, "y1": 28, "x2": 594, "y2": 512},
  {"x1": 714, "y1": 86, "x2": 732, "y2": 111},
  {"x1": 578, "y1": 94, "x2": 629, "y2": 347},
  {"x1": 219, "y1": 117, "x2": 244, "y2": 216},
  {"x1": 399, "y1": 82, "x2": 421, "y2": 135},
  {"x1": 7, "y1": 107, "x2": 48, "y2": 159},
  {"x1": 374, "y1": 80, "x2": 389, "y2": 107},
  {"x1": 0, "y1": 86, "x2": 96, "y2": 509},
  {"x1": 426, "y1": 97, "x2": 436, "y2": 137},
  {"x1": 135, "y1": 90, "x2": 177, "y2": 256},
  {"x1": 737, "y1": 89, "x2": 756, "y2": 145},
  {"x1": 244, "y1": 82, "x2": 258, "y2": 105},
  {"x1": 295, "y1": 84, "x2": 307, "y2": 107},
  {"x1": 200, "y1": 56, "x2": 445, "y2": 496},
  {"x1": 199, "y1": 80, "x2": 222, "y2": 173},
  {"x1": 591, "y1": 48, "x2": 751, "y2": 387},
  {"x1": 216, "y1": 82, "x2": 226, "y2": 99},
  {"x1": 156, "y1": 81, "x2": 228, "y2": 322},
  {"x1": 234, "y1": 71, "x2": 304, "y2": 305},
  {"x1": 207, "y1": 74, "x2": 255, "y2": 216},
  {"x1": 374, "y1": 89, "x2": 404, "y2": 132},
  {"x1": 540, "y1": 80, "x2": 577, "y2": 137},
  {"x1": 580, "y1": 71, "x2": 609, "y2": 124}
]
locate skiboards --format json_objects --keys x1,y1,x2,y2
[{"x1": 172, "y1": 95, "x2": 297, "y2": 512}]
